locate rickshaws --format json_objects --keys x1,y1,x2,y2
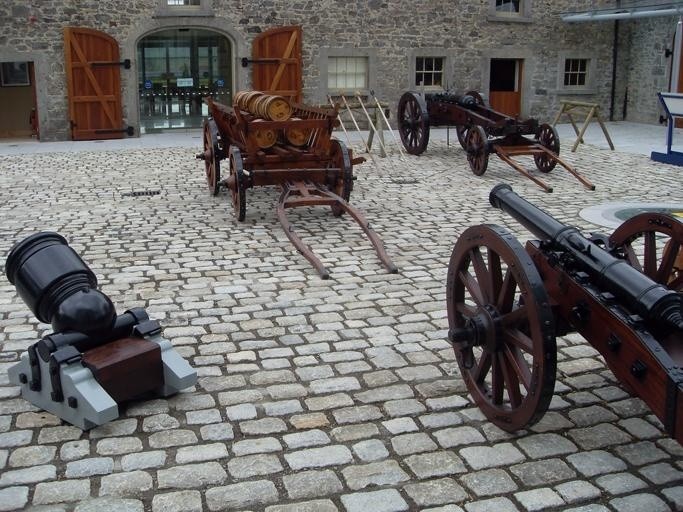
[
  {"x1": 397, "y1": 86, "x2": 597, "y2": 197},
  {"x1": 194, "y1": 93, "x2": 402, "y2": 281}
]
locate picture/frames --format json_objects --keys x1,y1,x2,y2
[{"x1": 1, "y1": 62, "x2": 31, "y2": 87}]
